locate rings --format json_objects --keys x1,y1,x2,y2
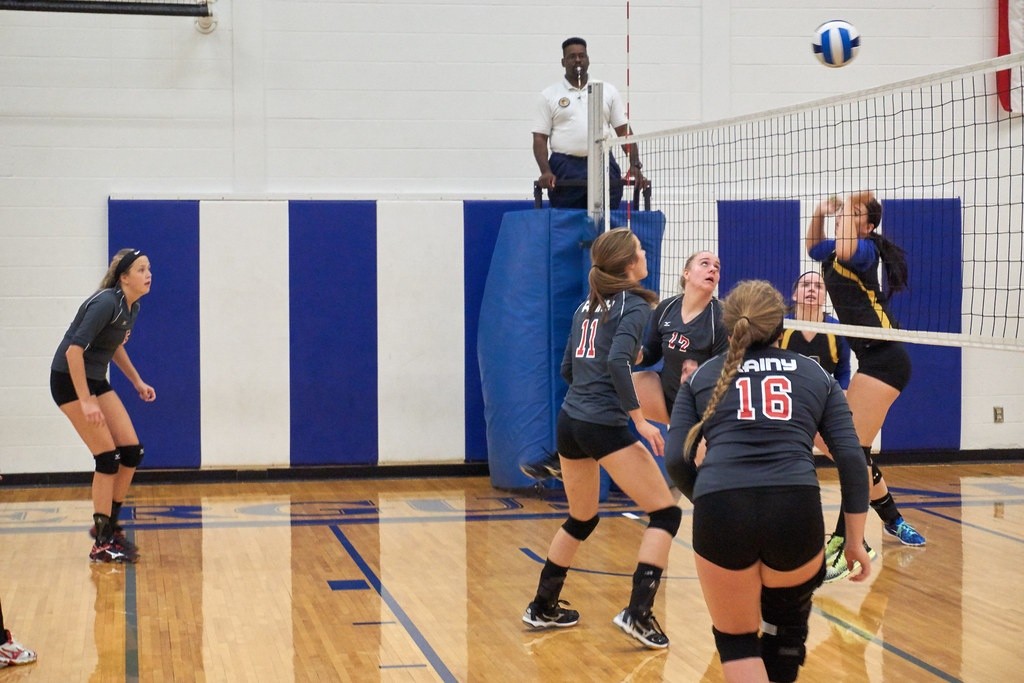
[{"x1": 643, "y1": 178, "x2": 647, "y2": 181}]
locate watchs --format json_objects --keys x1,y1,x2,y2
[{"x1": 630, "y1": 161, "x2": 643, "y2": 169}]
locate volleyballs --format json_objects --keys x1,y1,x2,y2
[{"x1": 810, "y1": 19, "x2": 860, "y2": 68}]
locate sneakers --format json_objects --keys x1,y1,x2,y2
[
  {"x1": 90, "y1": 522, "x2": 140, "y2": 564},
  {"x1": 824, "y1": 531, "x2": 878, "y2": 583},
  {"x1": 520, "y1": 445, "x2": 562, "y2": 486},
  {"x1": 523, "y1": 600, "x2": 580, "y2": 629},
  {"x1": 0, "y1": 629, "x2": 37, "y2": 668},
  {"x1": 884, "y1": 516, "x2": 927, "y2": 547},
  {"x1": 612, "y1": 606, "x2": 669, "y2": 649}
]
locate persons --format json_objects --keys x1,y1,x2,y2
[
  {"x1": 522, "y1": 226, "x2": 682, "y2": 649},
  {"x1": 664, "y1": 279, "x2": 870, "y2": 683},
  {"x1": 806, "y1": 192, "x2": 912, "y2": 583},
  {"x1": 50, "y1": 249, "x2": 156, "y2": 563},
  {"x1": 520, "y1": 251, "x2": 731, "y2": 482},
  {"x1": 0, "y1": 601, "x2": 38, "y2": 669},
  {"x1": 532, "y1": 38, "x2": 649, "y2": 211},
  {"x1": 770, "y1": 271, "x2": 926, "y2": 545}
]
